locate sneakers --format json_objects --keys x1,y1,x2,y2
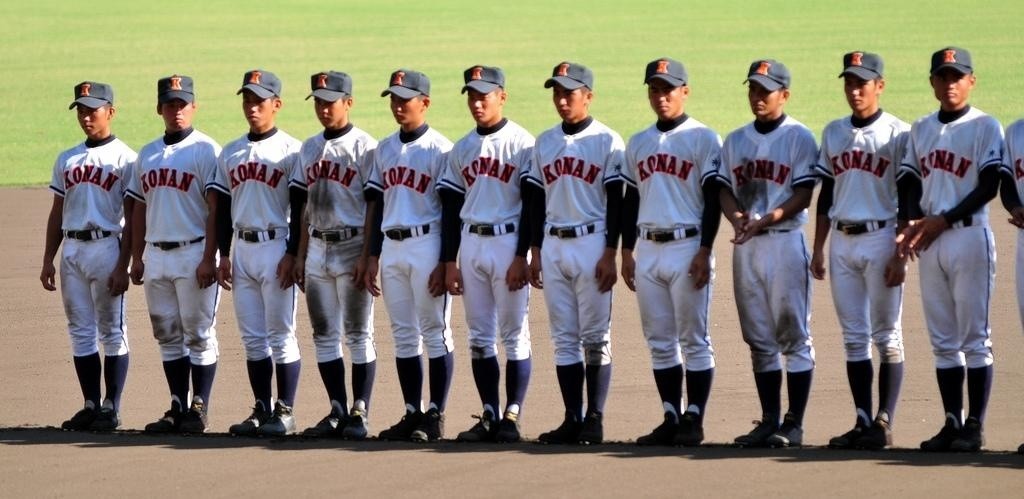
[
  {"x1": 457, "y1": 410, "x2": 520, "y2": 447},
  {"x1": 539, "y1": 411, "x2": 603, "y2": 448},
  {"x1": 378, "y1": 407, "x2": 446, "y2": 444},
  {"x1": 828, "y1": 419, "x2": 893, "y2": 452},
  {"x1": 341, "y1": 410, "x2": 368, "y2": 440},
  {"x1": 229, "y1": 400, "x2": 273, "y2": 438},
  {"x1": 766, "y1": 413, "x2": 803, "y2": 449},
  {"x1": 303, "y1": 408, "x2": 341, "y2": 441},
  {"x1": 734, "y1": 419, "x2": 776, "y2": 447},
  {"x1": 636, "y1": 412, "x2": 705, "y2": 449},
  {"x1": 258, "y1": 399, "x2": 298, "y2": 440},
  {"x1": 62, "y1": 406, "x2": 211, "y2": 436},
  {"x1": 920, "y1": 417, "x2": 985, "y2": 454}
]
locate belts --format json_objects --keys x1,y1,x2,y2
[
  {"x1": 239, "y1": 230, "x2": 275, "y2": 242},
  {"x1": 754, "y1": 228, "x2": 790, "y2": 236},
  {"x1": 462, "y1": 222, "x2": 514, "y2": 236},
  {"x1": 152, "y1": 236, "x2": 204, "y2": 250},
  {"x1": 312, "y1": 227, "x2": 356, "y2": 242},
  {"x1": 386, "y1": 224, "x2": 429, "y2": 241},
  {"x1": 638, "y1": 228, "x2": 697, "y2": 242},
  {"x1": 550, "y1": 225, "x2": 594, "y2": 238},
  {"x1": 837, "y1": 220, "x2": 885, "y2": 234},
  {"x1": 962, "y1": 213, "x2": 972, "y2": 228},
  {"x1": 67, "y1": 230, "x2": 110, "y2": 241}
]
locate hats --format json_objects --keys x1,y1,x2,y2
[
  {"x1": 306, "y1": 71, "x2": 352, "y2": 102},
  {"x1": 462, "y1": 65, "x2": 504, "y2": 93},
  {"x1": 544, "y1": 62, "x2": 593, "y2": 91},
  {"x1": 839, "y1": 51, "x2": 883, "y2": 81},
  {"x1": 381, "y1": 70, "x2": 431, "y2": 99},
  {"x1": 237, "y1": 70, "x2": 282, "y2": 99},
  {"x1": 743, "y1": 59, "x2": 790, "y2": 90},
  {"x1": 645, "y1": 58, "x2": 687, "y2": 87},
  {"x1": 70, "y1": 81, "x2": 113, "y2": 110},
  {"x1": 931, "y1": 47, "x2": 972, "y2": 75},
  {"x1": 158, "y1": 75, "x2": 194, "y2": 104}
]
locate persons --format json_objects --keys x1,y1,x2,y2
[
  {"x1": 716, "y1": 59, "x2": 820, "y2": 449},
  {"x1": 131, "y1": 75, "x2": 223, "y2": 434},
  {"x1": 40, "y1": 81, "x2": 138, "y2": 431},
  {"x1": 441, "y1": 64, "x2": 536, "y2": 444},
  {"x1": 293, "y1": 70, "x2": 379, "y2": 440},
  {"x1": 526, "y1": 60, "x2": 625, "y2": 445},
  {"x1": 364, "y1": 70, "x2": 455, "y2": 443},
  {"x1": 999, "y1": 119, "x2": 1024, "y2": 459},
  {"x1": 895, "y1": 46, "x2": 1003, "y2": 453},
  {"x1": 811, "y1": 52, "x2": 913, "y2": 450},
  {"x1": 621, "y1": 58, "x2": 723, "y2": 445},
  {"x1": 214, "y1": 69, "x2": 307, "y2": 439}
]
